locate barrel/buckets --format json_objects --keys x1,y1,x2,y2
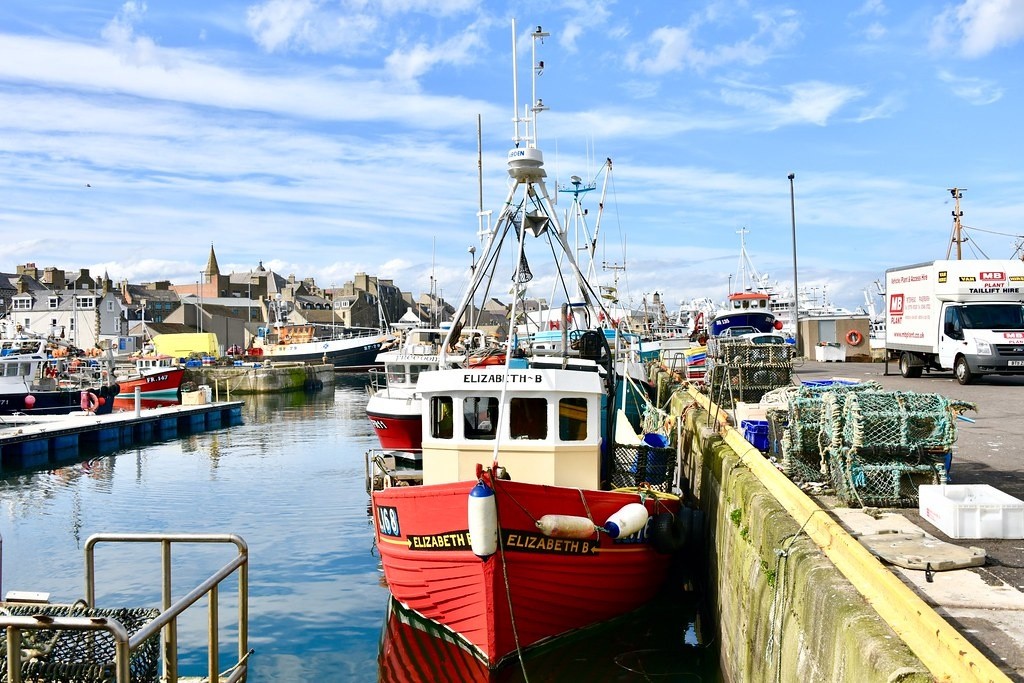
[{"x1": 632, "y1": 433, "x2": 669, "y2": 482}]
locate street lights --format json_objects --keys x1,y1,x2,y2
[{"x1": 787, "y1": 171, "x2": 802, "y2": 357}]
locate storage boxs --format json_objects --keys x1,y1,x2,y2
[
  {"x1": 685, "y1": 334, "x2": 980, "y2": 509},
  {"x1": 917, "y1": 482, "x2": 1024, "y2": 539},
  {"x1": 0, "y1": 600, "x2": 161, "y2": 683}
]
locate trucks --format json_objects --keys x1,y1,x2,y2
[{"x1": 886, "y1": 260, "x2": 1024, "y2": 386}]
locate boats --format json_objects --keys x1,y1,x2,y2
[
  {"x1": 1, "y1": 274, "x2": 188, "y2": 416},
  {"x1": 685, "y1": 225, "x2": 884, "y2": 345},
  {"x1": 244, "y1": 278, "x2": 404, "y2": 373},
  {"x1": 364, "y1": 19, "x2": 686, "y2": 669}
]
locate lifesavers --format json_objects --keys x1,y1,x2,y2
[
  {"x1": 85, "y1": 392, "x2": 99, "y2": 412},
  {"x1": 466, "y1": 353, "x2": 506, "y2": 365},
  {"x1": 846, "y1": 329, "x2": 862, "y2": 346}
]
